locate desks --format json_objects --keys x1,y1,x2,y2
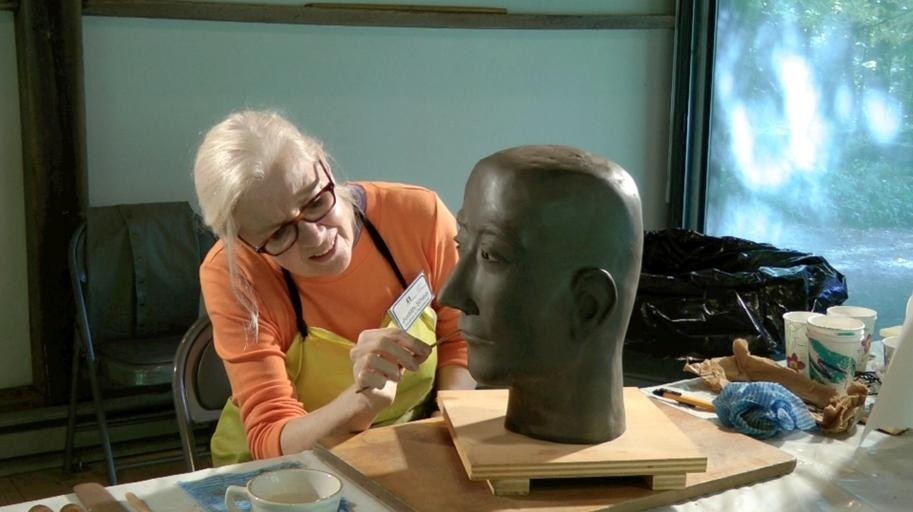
[{"x1": 1, "y1": 341, "x2": 913, "y2": 512}]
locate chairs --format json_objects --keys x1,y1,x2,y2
[
  {"x1": 61, "y1": 202, "x2": 220, "y2": 487},
  {"x1": 172, "y1": 313, "x2": 232, "y2": 477}
]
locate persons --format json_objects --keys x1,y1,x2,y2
[
  {"x1": 433, "y1": 144, "x2": 645, "y2": 446},
  {"x1": 191, "y1": 108, "x2": 475, "y2": 477}
]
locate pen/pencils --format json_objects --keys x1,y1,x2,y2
[{"x1": 653, "y1": 388, "x2": 715, "y2": 414}]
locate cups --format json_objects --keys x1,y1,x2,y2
[
  {"x1": 224, "y1": 467, "x2": 344, "y2": 512},
  {"x1": 782, "y1": 310, "x2": 826, "y2": 376},
  {"x1": 827, "y1": 305, "x2": 878, "y2": 371},
  {"x1": 806, "y1": 314, "x2": 866, "y2": 393}
]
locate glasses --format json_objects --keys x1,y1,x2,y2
[{"x1": 236, "y1": 155, "x2": 336, "y2": 257}]
